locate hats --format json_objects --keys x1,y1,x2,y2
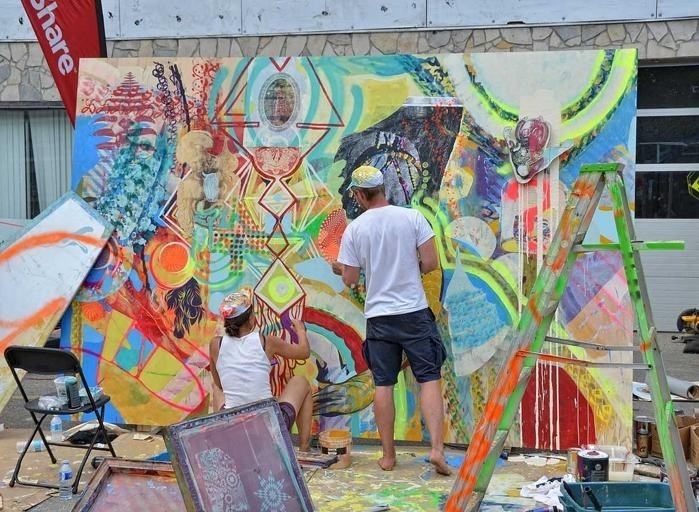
[
  {"x1": 220, "y1": 285, "x2": 254, "y2": 318},
  {"x1": 345, "y1": 165, "x2": 384, "y2": 191}
]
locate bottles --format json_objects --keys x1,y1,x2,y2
[
  {"x1": 15, "y1": 415, "x2": 62, "y2": 452},
  {"x1": 635, "y1": 429, "x2": 649, "y2": 457},
  {"x1": 60, "y1": 460, "x2": 73, "y2": 499}
]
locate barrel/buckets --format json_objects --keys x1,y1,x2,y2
[
  {"x1": 319, "y1": 430, "x2": 353, "y2": 470},
  {"x1": 578, "y1": 448, "x2": 610, "y2": 484}
]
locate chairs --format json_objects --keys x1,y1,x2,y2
[{"x1": 4, "y1": 345, "x2": 119, "y2": 494}]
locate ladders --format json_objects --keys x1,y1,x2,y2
[{"x1": 442, "y1": 161, "x2": 698, "y2": 511}]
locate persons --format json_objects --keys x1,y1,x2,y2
[
  {"x1": 206, "y1": 285, "x2": 318, "y2": 457},
  {"x1": 330, "y1": 164, "x2": 455, "y2": 479}
]
